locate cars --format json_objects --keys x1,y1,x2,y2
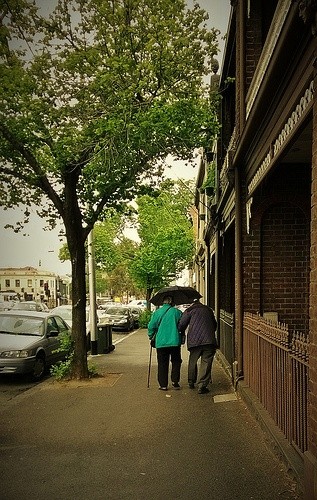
[
  {"x1": 0, "y1": 299, "x2": 50, "y2": 314},
  {"x1": 47, "y1": 305, "x2": 100, "y2": 352},
  {"x1": 0, "y1": 311, "x2": 72, "y2": 382},
  {"x1": 96, "y1": 297, "x2": 154, "y2": 310},
  {"x1": 101, "y1": 306, "x2": 134, "y2": 332}
]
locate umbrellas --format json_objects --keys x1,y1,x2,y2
[{"x1": 148, "y1": 286, "x2": 203, "y2": 305}]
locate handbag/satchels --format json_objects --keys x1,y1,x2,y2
[{"x1": 150, "y1": 335, "x2": 156, "y2": 348}]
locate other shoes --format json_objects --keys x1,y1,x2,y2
[
  {"x1": 189, "y1": 382, "x2": 194, "y2": 389},
  {"x1": 172, "y1": 382, "x2": 180, "y2": 389},
  {"x1": 198, "y1": 388, "x2": 209, "y2": 394},
  {"x1": 158, "y1": 386, "x2": 167, "y2": 391}
]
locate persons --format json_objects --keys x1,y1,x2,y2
[
  {"x1": 147, "y1": 296, "x2": 187, "y2": 390},
  {"x1": 177, "y1": 300, "x2": 218, "y2": 394}
]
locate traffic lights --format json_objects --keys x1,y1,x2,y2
[
  {"x1": 44, "y1": 283, "x2": 47, "y2": 291},
  {"x1": 45, "y1": 290, "x2": 49, "y2": 296}
]
